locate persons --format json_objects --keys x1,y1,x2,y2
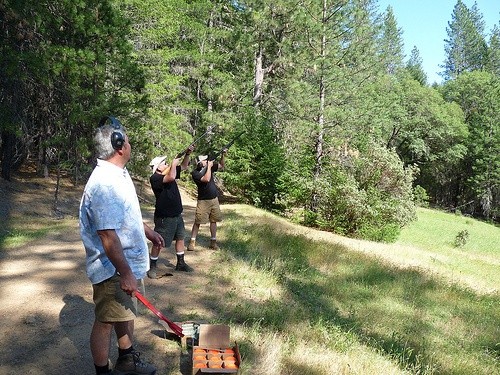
[
  {"x1": 147, "y1": 145, "x2": 194, "y2": 279},
  {"x1": 187, "y1": 148, "x2": 228, "y2": 251},
  {"x1": 80, "y1": 115, "x2": 166, "y2": 375}
]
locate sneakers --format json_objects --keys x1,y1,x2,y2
[{"x1": 113, "y1": 349, "x2": 156, "y2": 375}]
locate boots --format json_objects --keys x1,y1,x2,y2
[
  {"x1": 147, "y1": 258, "x2": 158, "y2": 279},
  {"x1": 175, "y1": 254, "x2": 194, "y2": 272}
]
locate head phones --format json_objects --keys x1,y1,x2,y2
[
  {"x1": 98, "y1": 116, "x2": 124, "y2": 150},
  {"x1": 195, "y1": 156, "x2": 203, "y2": 168}
]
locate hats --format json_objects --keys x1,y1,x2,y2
[
  {"x1": 149, "y1": 155, "x2": 167, "y2": 174},
  {"x1": 194, "y1": 155, "x2": 208, "y2": 166}
]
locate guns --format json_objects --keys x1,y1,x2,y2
[
  {"x1": 162, "y1": 125, "x2": 216, "y2": 175},
  {"x1": 195, "y1": 130, "x2": 246, "y2": 181}
]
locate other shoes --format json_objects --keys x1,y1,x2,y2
[
  {"x1": 187, "y1": 239, "x2": 196, "y2": 251},
  {"x1": 209, "y1": 239, "x2": 220, "y2": 251}
]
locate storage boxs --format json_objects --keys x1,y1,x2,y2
[{"x1": 158, "y1": 319, "x2": 243, "y2": 375}]
